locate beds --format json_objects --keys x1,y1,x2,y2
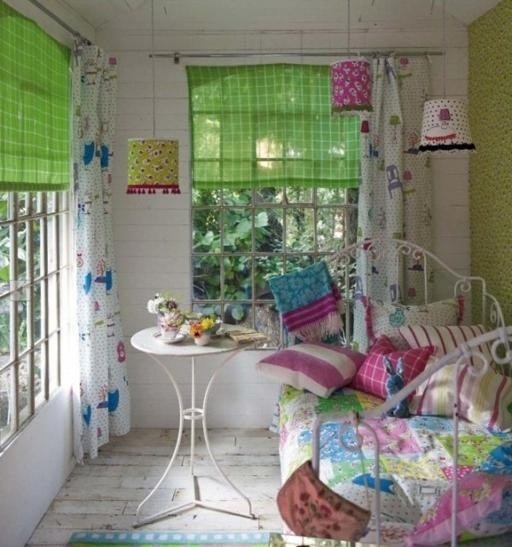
[{"x1": 268, "y1": 239, "x2": 512, "y2": 547}]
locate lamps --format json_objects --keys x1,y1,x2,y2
[
  {"x1": 414, "y1": 0, "x2": 478, "y2": 155},
  {"x1": 125, "y1": 0, "x2": 181, "y2": 194},
  {"x1": 331, "y1": 1, "x2": 374, "y2": 114}
]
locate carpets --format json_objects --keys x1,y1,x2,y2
[{"x1": 68, "y1": 530, "x2": 283, "y2": 547}]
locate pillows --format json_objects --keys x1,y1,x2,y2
[
  {"x1": 361, "y1": 296, "x2": 464, "y2": 354},
  {"x1": 349, "y1": 337, "x2": 435, "y2": 403},
  {"x1": 254, "y1": 343, "x2": 364, "y2": 399},
  {"x1": 397, "y1": 324, "x2": 496, "y2": 371},
  {"x1": 408, "y1": 361, "x2": 511, "y2": 434}
]
